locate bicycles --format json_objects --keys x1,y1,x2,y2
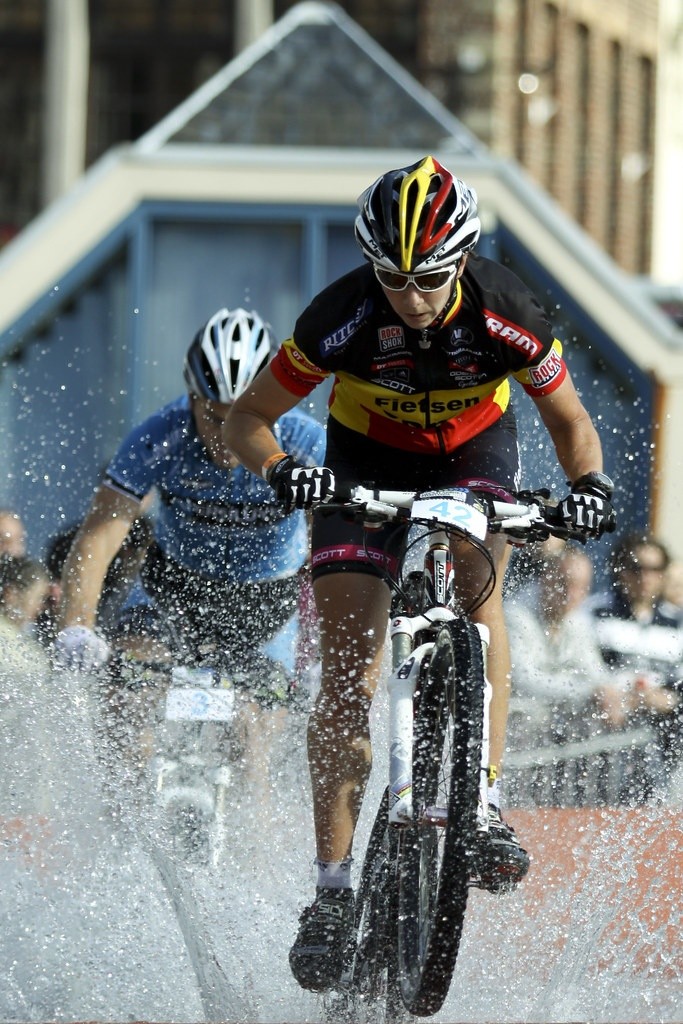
[
  {"x1": 103, "y1": 648, "x2": 313, "y2": 891},
  {"x1": 332, "y1": 477, "x2": 617, "y2": 1020}
]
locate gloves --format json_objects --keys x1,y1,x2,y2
[
  {"x1": 268, "y1": 456, "x2": 336, "y2": 515},
  {"x1": 296, "y1": 669, "x2": 324, "y2": 712},
  {"x1": 54, "y1": 626, "x2": 109, "y2": 675},
  {"x1": 556, "y1": 473, "x2": 617, "y2": 540}
]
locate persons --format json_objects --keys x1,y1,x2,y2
[
  {"x1": 225, "y1": 154, "x2": 621, "y2": 989},
  {"x1": 1, "y1": 509, "x2": 683, "y2": 741},
  {"x1": 2, "y1": 305, "x2": 333, "y2": 884}
]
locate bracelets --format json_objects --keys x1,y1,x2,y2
[{"x1": 261, "y1": 453, "x2": 288, "y2": 482}]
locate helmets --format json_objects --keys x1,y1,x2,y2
[
  {"x1": 182, "y1": 308, "x2": 273, "y2": 404},
  {"x1": 353, "y1": 155, "x2": 481, "y2": 273}
]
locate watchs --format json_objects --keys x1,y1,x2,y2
[{"x1": 570, "y1": 470, "x2": 615, "y2": 501}]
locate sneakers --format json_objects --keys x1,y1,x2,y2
[
  {"x1": 289, "y1": 885, "x2": 356, "y2": 988},
  {"x1": 468, "y1": 804, "x2": 529, "y2": 883}
]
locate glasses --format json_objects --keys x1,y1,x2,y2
[
  {"x1": 624, "y1": 561, "x2": 664, "y2": 574},
  {"x1": 371, "y1": 260, "x2": 459, "y2": 292}
]
